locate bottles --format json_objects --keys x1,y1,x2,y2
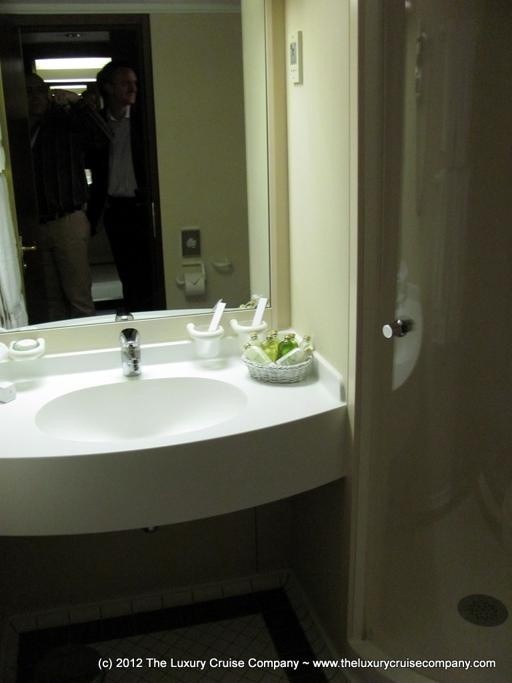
[
  {"x1": 9, "y1": 338, "x2": 45, "y2": 389},
  {"x1": 242, "y1": 328, "x2": 312, "y2": 367}
]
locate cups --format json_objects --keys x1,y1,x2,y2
[
  {"x1": 187, "y1": 322, "x2": 224, "y2": 365},
  {"x1": 232, "y1": 318, "x2": 267, "y2": 352}
]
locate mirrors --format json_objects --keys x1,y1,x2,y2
[{"x1": 0, "y1": 0, "x2": 272, "y2": 335}]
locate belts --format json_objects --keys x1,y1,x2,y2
[{"x1": 38, "y1": 205, "x2": 83, "y2": 221}]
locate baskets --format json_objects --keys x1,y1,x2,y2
[{"x1": 241, "y1": 355, "x2": 313, "y2": 383}]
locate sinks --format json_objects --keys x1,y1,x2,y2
[{"x1": 34, "y1": 376, "x2": 248, "y2": 445}]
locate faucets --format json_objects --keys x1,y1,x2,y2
[
  {"x1": 118, "y1": 327, "x2": 142, "y2": 377},
  {"x1": 115, "y1": 307, "x2": 134, "y2": 323}
]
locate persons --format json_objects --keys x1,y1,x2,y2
[
  {"x1": 29, "y1": 70, "x2": 97, "y2": 324},
  {"x1": 79, "y1": 58, "x2": 168, "y2": 313}
]
